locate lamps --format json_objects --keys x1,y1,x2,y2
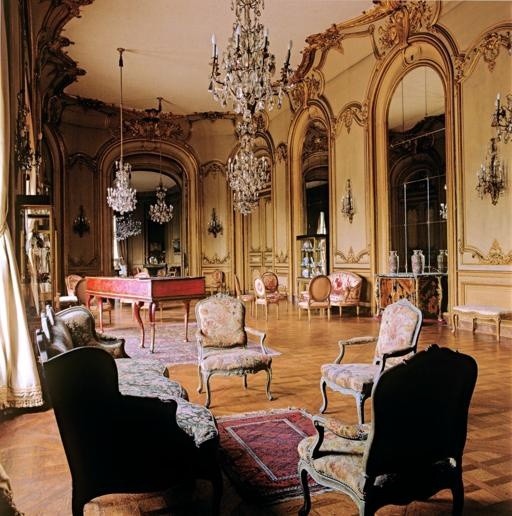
[
  {"x1": 112, "y1": 211, "x2": 143, "y2": 242},
  {"x1": 207, "y1": 0, "x2": 295, "y2": 217},
  {"x1": 105, "y1": 48, "x2": 136, "y2": 217},
  {"x1": 473, "y1": 137, "x2": 506, "y2": 205},
  {"x1": 148, "y1": 97, "x2": 173, "y2": 223},
  {"x1": 340, "y1": 177, "x2": 356, "y2": 222},
  {"x1": 490, "y1": 90, "x2": 512, "y2": 143},
  {"x1": 73, "y1": 204, "x2": 89, "y2": 235},
  {"x1": 205, "y1": 209, "x2": 222, "y2": 237}
]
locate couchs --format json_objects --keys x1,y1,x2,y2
[
  {"x1": 37, "y1": 304, "x2": 227, "y2": 515},
  {"x1": 326, "y1": 272, "x2": 363, "y2": 318}
]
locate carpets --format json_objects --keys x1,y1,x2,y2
[
  {"x1": 214, "y1": 406, "x2": 348, "y2": 513},
  {"x1": 99, "y1": 321, "x2": 283, "y2": 369}
]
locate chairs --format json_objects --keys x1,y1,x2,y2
[
  {"x1": 298, "y1": 274, "x2": 331, "y2": 321},
  {"x1": 195, "y1": 293, "x2": 273, "y2": 409},
  {"x1": 296, "y1": 344, "x2": 478, "y2": 516},
  {"x1": 319, "y1": 298, "x2": 423, "y2": 424},
  {"x1": 254, "y1": 277, "x2": 280, "y2": 320},
  {"x1": 56, "y1": 274, "x2": 82, "y2": 310},
  {"x1": 259, "y1": 271, "x2": 289, "y2": 316},
  {"x1": 206, "y1": 270, "x2": 227, "y2": 296},
  {"x1": 233, "y1": 273, "x2": 256, "y2": 317}
]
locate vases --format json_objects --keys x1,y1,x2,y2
[{"x1": 412, "y1": 247, "x2": 426, "y2": 272}]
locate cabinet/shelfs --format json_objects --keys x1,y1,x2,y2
[{"x1": 294, "y1": 232, "x2": 329, "y2": 283}]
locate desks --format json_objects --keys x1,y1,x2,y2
[{"x1": 83, "y1": 275, "x2": 205, "y2": 353}]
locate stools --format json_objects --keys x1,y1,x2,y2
[{"x1": 449, "y1": 303, "x2": 512, "y2": 344}]
